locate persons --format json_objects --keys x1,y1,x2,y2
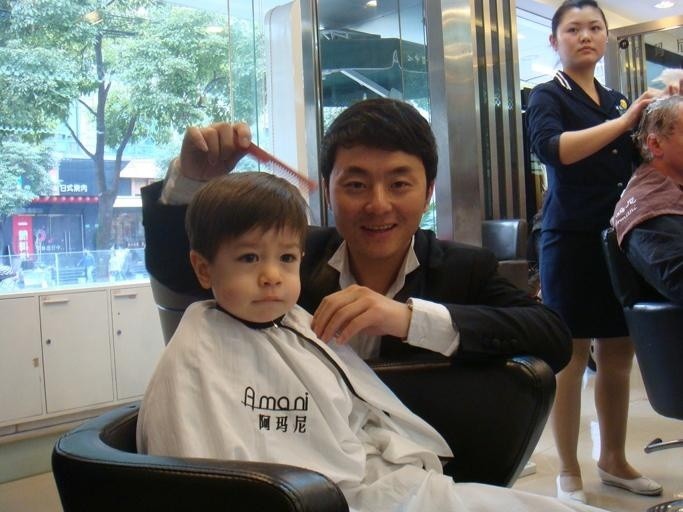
[
  {"x1": 526, "y1": 0, "x2": 683, "y2": 507},
  {"x1": 613, "y1": 93, "x2": 683, "y2": 308},
  {"x1": 133, "y1": 170, "x2": 614, "y2": 511},
  {"x1": 0, "y1": 223, "x2": 145, "y2": 291},
  {"x1": 140, "y1": 96, "x2": 575, "y2": 378}
]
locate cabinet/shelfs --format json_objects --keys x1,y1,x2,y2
[{"x1": 0, "y1": 279, "x2": 165, "y2": 428}]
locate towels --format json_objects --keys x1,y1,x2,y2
[{"x1": 609, "y1": 163, "x2": 683, "y2": 251}]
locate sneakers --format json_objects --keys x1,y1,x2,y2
[
  {"x1": 556, "y1": 473, "x2": 587, "y2": 504},
  {"x1": 598, "y1": 462, "x2": 664, "y2": 497}
]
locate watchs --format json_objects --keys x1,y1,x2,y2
[{"x1": 400, "y1": 301, "x2": 413, "y2": 346}]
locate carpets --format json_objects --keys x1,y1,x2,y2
[{"x1": 0, "y1": 430, "x2": 72, "y2": 484}]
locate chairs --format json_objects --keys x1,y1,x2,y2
[
  {"x1": 52, "y1": 354, "x2": 556, "y2": 512},
  {"x1": 601, "y1": 229, "x2": 683, "y2": 512},
  {"x1": 483, "y1": 220, "x2": 528, "y2": 291}
]
